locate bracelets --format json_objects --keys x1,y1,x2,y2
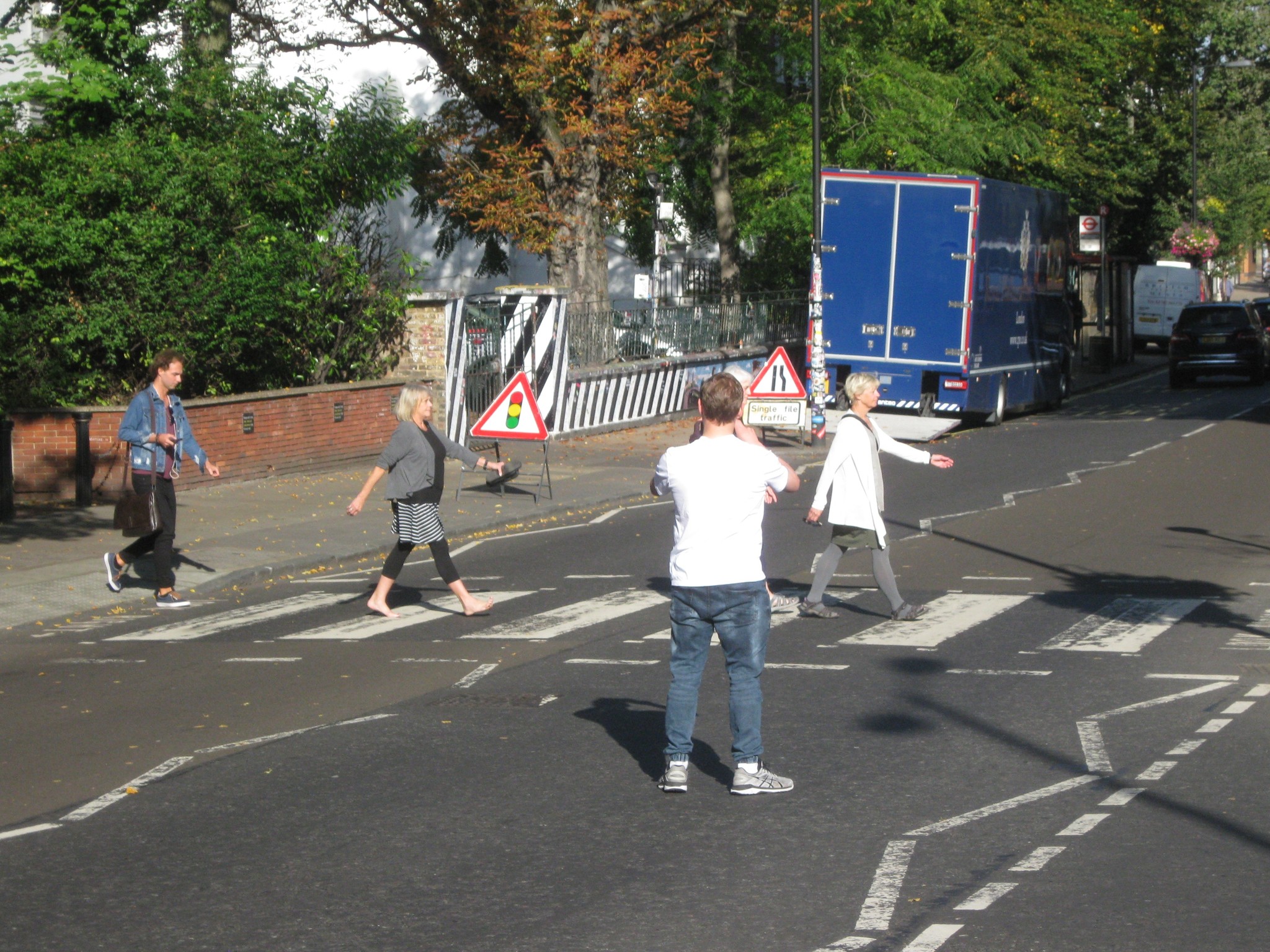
[
  {"x1": 156, "y1": 432, "x2": 162, "y2": 443},
  {"x1": 929, "y1": 453, "x2": 932, "y2": 463},
  {"x1": 483, "y1": 459, "x2": 489, "y2": 470}
]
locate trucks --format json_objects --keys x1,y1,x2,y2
[{"x1": 803, "y1": 163, "x2": 1086, "y2": 426}]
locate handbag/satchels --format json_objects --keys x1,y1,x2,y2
[{"x1": 113, "y1": 489, "x2": 163, "y2": 537}]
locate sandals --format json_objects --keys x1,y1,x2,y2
[
  {"x1": 890, "y1": 602, "x2": 929, "y2": 621},
  {"x1": 770, "y1": 593, "x2": 799, "y2": 612},
  {"x1": 799, "y1": 597, "x2": 840, "y2": 618}
]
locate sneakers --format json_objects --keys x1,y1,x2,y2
[
  {"x1": 730, "y1": 759, "x2": 793, "y2": 796},
  {"x1": 104, "y1": 552, "x2": 121, "y2": 591},
  {"x1": 156, "y1": 591, "x2": 190, "y2": 608},
  {"x1": 657, "y1": 762, "x2": 689, "y2": 792}
]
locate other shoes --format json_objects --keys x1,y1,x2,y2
[{"x1": 486, "y1": 460, "x2": 521, "y2": 487}]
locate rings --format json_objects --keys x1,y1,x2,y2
[
  {"x1": 349, "y1": 505, "x2": 351, "y2": 508},
  {"x1": 350, "y1": 508, "x2": 352, "y2": 510}
]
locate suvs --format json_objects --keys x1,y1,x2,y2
[{"x1": 1167, "y1": 297, "x2": 1270, "y2": 391}]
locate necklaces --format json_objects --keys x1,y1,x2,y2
[{"x1": 166, "y1": 410, "x2": 171, "y2": 420}]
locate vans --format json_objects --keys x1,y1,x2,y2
[{"x1": 1131, "y1": 263, "x2": 1213, "y2": 352}]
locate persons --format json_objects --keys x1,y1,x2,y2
[
  {"x1": 1071, "y1": 289, "x2": 1087, "y2": 351},
  {"x1": 797, "y1": 372, "x2": 954, "y2": 621},
  {"x1": 103, "y1": 349, "x2": 220, "y2": 607},
  {"x1": 346, "y1": 383, "x2": 504, "y2": 618},
  {"x1": 1220, "y1": 274, "x2": 1233, "y2": 303},
  {"x1": 723, "y1": 365, "x2": 800, "y2": 612},
  {"x1": 650, "y1": 371, "x2": 800, "y2": 795}
]
